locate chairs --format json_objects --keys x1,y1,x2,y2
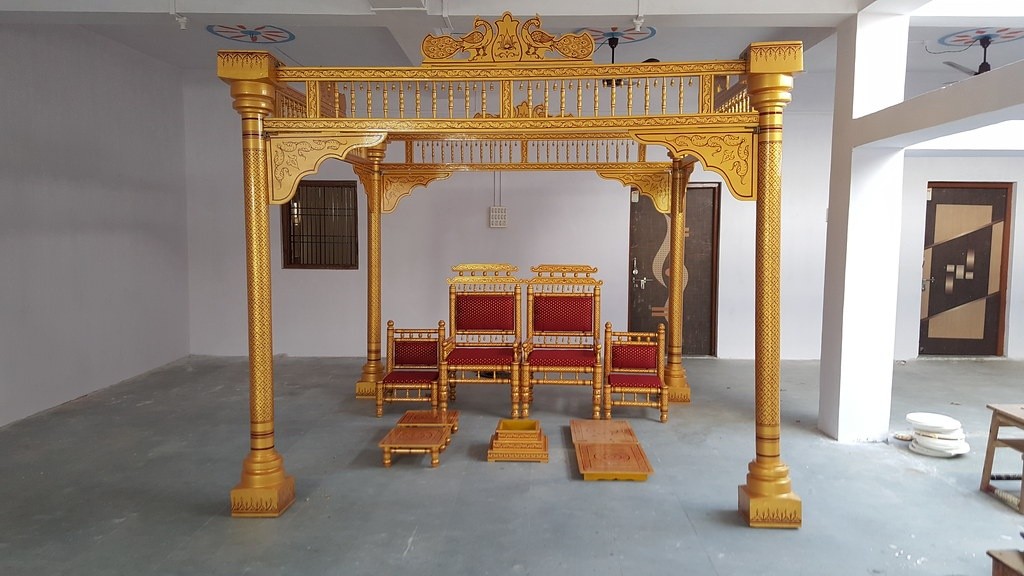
[
  {"x1": 439, "y1": 263, "x2": 520, "y2": 419},
  {"x1": 375, "y1": 320, "x2": 447, "y2": 417},
  {"x1": 522, "y1": 264, "x2": 603, "y2": 420},
  {"x1": 604, "y1": 322, "x2": 668, "y2": 424}
]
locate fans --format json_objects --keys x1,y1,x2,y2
[
  {"x1": 942, "y1": 36, "x2": 992, "y2": 85},
  {"x1": 602, "y1": 37, "x2": 661, "y2": 89}
]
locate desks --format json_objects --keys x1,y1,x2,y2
[{"x1": 978, "y1": 402, "x2": 1024, "y2": 515}]
